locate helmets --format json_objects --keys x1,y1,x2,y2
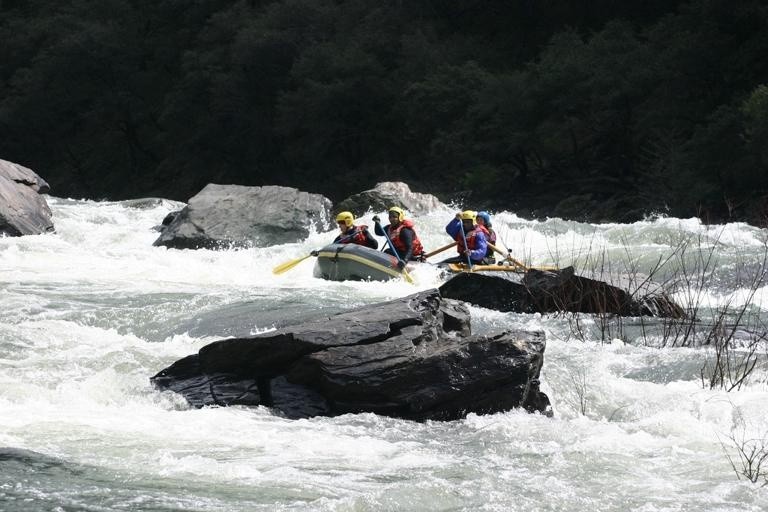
[
  {"x1": 388, "y1": 206, "x2": 404, "y2": 223},
  {"x1": 335, "y1": 211, "x2": 354, "y2": 227},
  {"x1": 461, "y1": 210, "x2": 490, "y2": 225}
]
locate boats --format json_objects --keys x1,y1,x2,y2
[{"x1": 314, "y1": 241, "x2": 560, "y2": 284}]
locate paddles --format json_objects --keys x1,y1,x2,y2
[
  {"x1": 376, "y1": 219, "x2": 415, "y2": 284},
  {"x1": 271, "y1": 229, "x2": 362, "y2": 275},
  {"x1": 449, "y1": 263, "x2": 554, "y2": 272}
]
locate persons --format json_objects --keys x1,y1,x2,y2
[
  {"x1": 442, "y1": 210, "x2": 496, "y2": 264},
  {"x1": 372, "y1": 207, "x2": 426, "y2": 262},
  {"x1": 334, "y1": 211, "x2": 378, "y2": 249}
]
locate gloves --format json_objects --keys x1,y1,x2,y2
[
  {"x1": 311, "y1": 249, "x2": 319, "y2": 256},
  {"x1": 455, "y1": 212, "x2": 462, "y2": 220},
  {"x1": 372, "y1": 215, "x2": 381, "y2": 223},
  {"x1": 397, "y1": 260, "x2": 406, "y2": 270},
  {"x1": 463, "y1": 249, "x2": 471, "y2": 257}
]
